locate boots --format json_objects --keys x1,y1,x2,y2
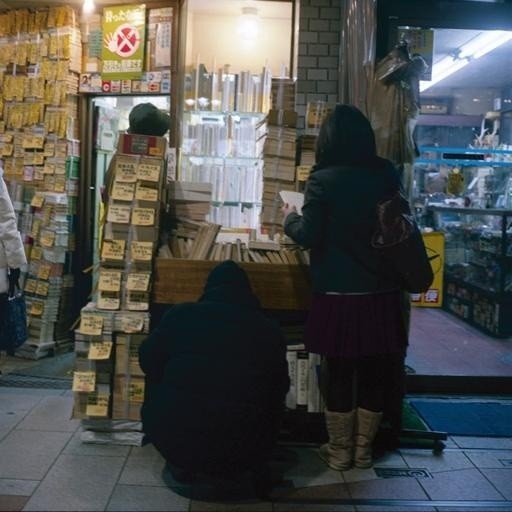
[
  {"x1": 318, "y1": 407, "x2": 356, "y2": 472},
  {"x1": 353, "y1": 406, "x2": 383, "y2": 469}
]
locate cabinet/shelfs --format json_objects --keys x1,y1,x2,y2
[{"x1": 426, "y1": 203, "x2": 511, "y2": 338}]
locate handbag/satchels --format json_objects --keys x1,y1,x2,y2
[
  {"x1": 0, "y1": 280, "x2": 27, "y2": 350},
  {"x1": 370, "y1": 191, "x2": 434, "y2": 294}
]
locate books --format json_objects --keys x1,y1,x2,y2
[
  {"x1": 156, "y1": 65, "x2": 314, "y2": 265},
  {"x1": 287, "y1": 343, "x2": 330, "y2": 413}
]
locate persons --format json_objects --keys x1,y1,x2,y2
[
  {"x1": 278, "y1": 102, "x2": 409, "y2": 470},
  {"x1": 137, "y1": 260, "x2": 289, "y2": 504},
  {"x1": 1, "y1": 165, "x2": 29, "y2": 345},
  {"x1": 98, "y1": 103, "x2": 175, "y2": 261}
]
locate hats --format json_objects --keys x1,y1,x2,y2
[{"x1": 127, "y1": 102, "x2": 172, "y2": 138}]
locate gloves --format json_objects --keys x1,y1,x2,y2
[{"x1": 9, "y1": 267, "x2": 21, "y2": 282}]
